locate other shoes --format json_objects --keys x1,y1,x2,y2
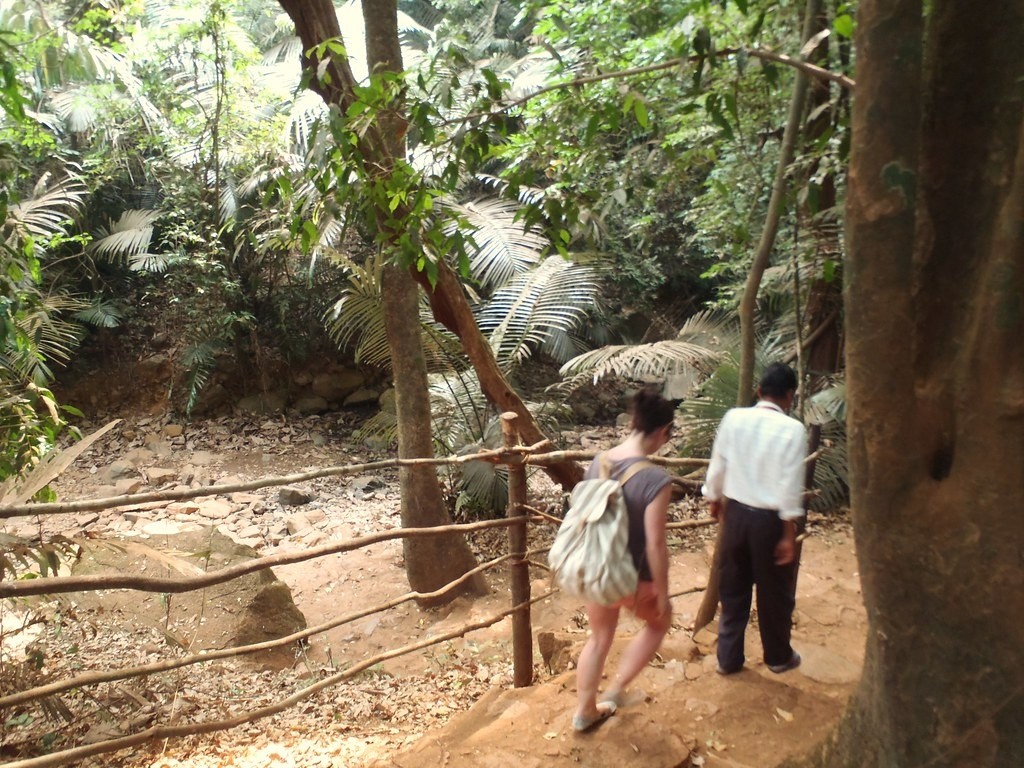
[
  {"x1": 769, "y1": 651, "x2": 801, "y2": 673},
  {"x1": 717, "y1": 663, "x2": 743, "y2": 674},
  {"x1": 573, "y1": 700, "x2": 619, "y2": 732},
  {"x1": 605, "y1": 687, "x2": 646, "y2": 709}
]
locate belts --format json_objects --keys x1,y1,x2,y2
[{"x1": 730, "y1": 499, "x2": 779, "y2": 515}]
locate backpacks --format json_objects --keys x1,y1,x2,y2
[{"x1": 548, "y1": 454, "x2": 652, "y2": 605}]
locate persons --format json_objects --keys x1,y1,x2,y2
[
  {"x1": 573, "y1": 395, "x2": 673, "y2": 732},
  {"x1": 699, "y1": 363, "x2": 803, "y2": 674}
]
